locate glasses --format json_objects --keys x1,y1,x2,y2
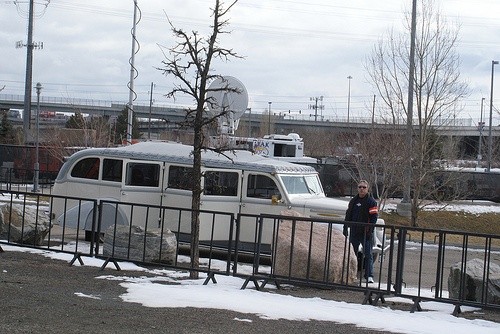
[{"x1": 358, "y1": 186, "x2": 367, "y2": 189}]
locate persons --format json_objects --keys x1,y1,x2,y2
[{"x1": 343, "y1": 179, "x2": 381, "y2": 284}]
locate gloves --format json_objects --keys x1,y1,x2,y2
[
  {"x1": 343, "y1": 227, "x2": 348, "y2": 237},
  {"x1": 366, "y1": 231, "x2": 372, "y2": 240}
]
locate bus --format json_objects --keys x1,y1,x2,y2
[{"x1": 48, "y1": 140, "x2": 389, "y2": 275}]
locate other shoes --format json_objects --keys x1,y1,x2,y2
[{"x1": 368, "y1": 276, "x2": 374, "y2": 283}]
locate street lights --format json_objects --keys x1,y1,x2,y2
[
  {"x1": 267, "y1": 101, "x2": 272, "y2": 135},
  {"x1": 487, "y1": 59, "x2": 500, "y2": 172},
  {"x1": 346, "y1": 75, "x2": 352, "y2": 123},
  {"x1": 31, "y1": 82, "x2": 44, "y2": 192}
]
